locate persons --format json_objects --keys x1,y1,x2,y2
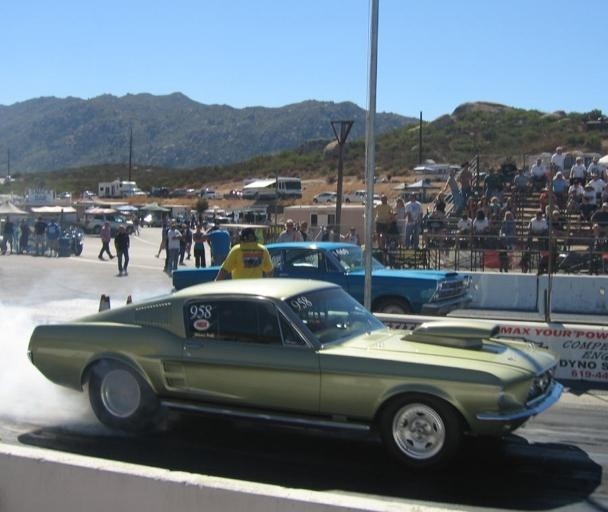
[
  {"x1": 98, "y1": 221, "x2": 115, "y2": 261},
  {"x1": 2, "y1": 215, "x2": 61, "y2": 257},
  {"x1": 114, "y1": 224, "x2": 131, "y2": 276},
  {"x1": 154, "y1": 213, "x2": 230, "y2": 276},
  {"x1": 276, "y1": 218, "x2": 362, "y2": 248},
  {"x1": 214, "y1": 227, "x2": 273, "y2": 281},
  {"x1": 372, "y1": 143, "x2": 608, "y2": 277}
]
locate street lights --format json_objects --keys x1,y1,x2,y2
[{"x1": 331, "y1": 118, "x2": 354, "y2": 241}]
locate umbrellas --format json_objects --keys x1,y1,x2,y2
[
  {"x1": 0, "y1": 199, "x2": 29, "y2": 216},
  {"x1": 116, "y1": 205, "x2": 139, "y2": 212}
]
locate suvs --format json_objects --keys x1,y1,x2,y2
[{"x1": 81, "y1": 208, "x2": 134, "y2": 236}]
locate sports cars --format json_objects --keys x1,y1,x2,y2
[{"x1": 27, "y1": 277, "x2": 565, "y2": 472}]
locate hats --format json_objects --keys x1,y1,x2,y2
[{"x1": 239, "y1": 229, "x2": 257, "y2": 241}]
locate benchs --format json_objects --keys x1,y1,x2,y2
[{"x1": 446, "y1": 185, "x2": 607, "y2": 234}]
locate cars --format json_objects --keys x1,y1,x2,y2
[
  {"x1": 310, "y1": 190, "x2": 381, "y2": 207},
  {"x1": 167, "y1": 188, "x2": 217, "y2": 200},
  {"x1": 140, "y1": 214, "x2": 233, "y2": 230},
  {"x1": 80, "y1": 190, "x2": 96, "y2": 200},
  {"x1": 60, "y1": 192, "x2": 72, "y2": 201},
  {"x1": 171, "y1": 241, "x2": 473, "y2": 318},
  {"x1": 123, "y1": 187, "x2": 146, "y2": 197}
]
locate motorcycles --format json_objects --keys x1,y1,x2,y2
[{"x1": 61, "y1": 226, "x2": 84, "y2": 258}]
